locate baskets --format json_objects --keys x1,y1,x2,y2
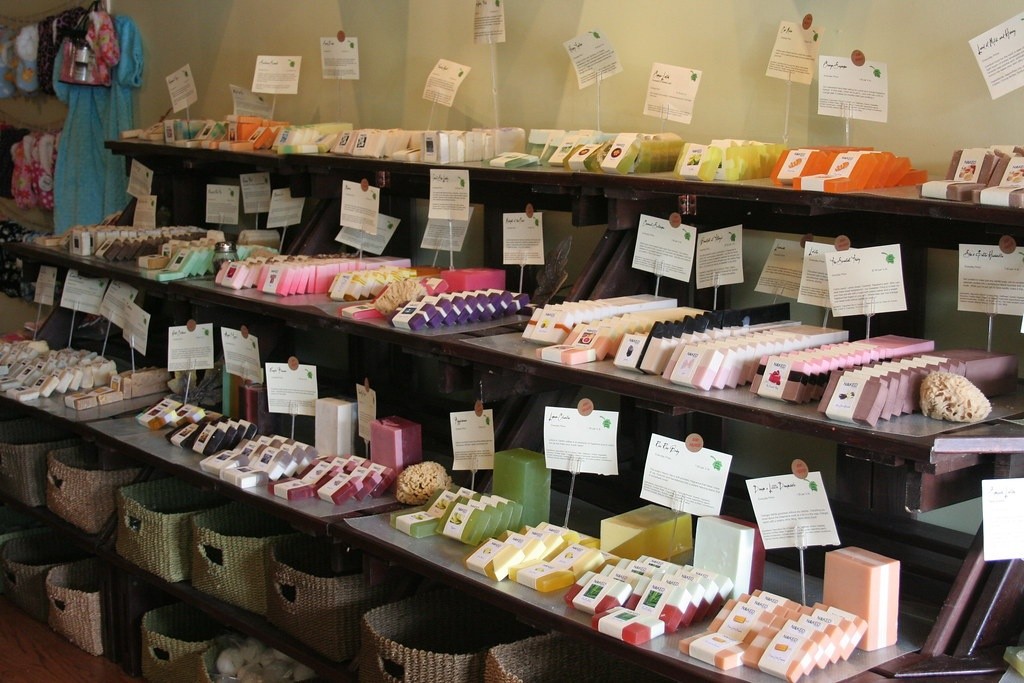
[
  {"x1": 484, "y1": 632, "x2": 677, "y2": 683},
  {"x1": 0, "y1": 419, "x2": 84, "y2": 507},
  {"x1": 45, "y1": 556, "x2": 139, "y2": 656},
  {"x1": 201, "y1": 635, "x2": 320, "y2": 683},
  {"x1": 0, "y1": 506, "x2": 52, "y2": 594},
  {"x1": 190, "y1": 501, "x2": 304, "y2": 615},
  {"x1": 47, "y1": 446, "x2": 144, "y2": 535},
  {"x1": 359, "y1": 589, "x2": 546, "y2": 683},
  {"x1": 0, "y1": 532, "x2": 96, "y2": 623},
  {"x1": 115, "y1": 476, "x2": 237, "y2": 584},
  {"x1": 0, "y1": 405, "x2": 30, "y2": 421},
  {"x1": 140, "y1": 603, "x2": 244, "y2": 683},
  {"x1": 267, "y1": 535, "x2": 422, "y2": 663}
]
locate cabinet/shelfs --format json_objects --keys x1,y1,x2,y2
[{"x1": 0, "y1": 139, "x2": 1024, "y2": 683}]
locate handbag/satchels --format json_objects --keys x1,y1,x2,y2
[{"x1": 57, "y1": 1, "x2": 112, "y2": 87}]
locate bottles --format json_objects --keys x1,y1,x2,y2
[
  {"x1": 73, "y1": 41, "x2": 92, "y2": 82},
  {"x1": 212, "y1": 242, "x2": 238, "y2": 275}
]
